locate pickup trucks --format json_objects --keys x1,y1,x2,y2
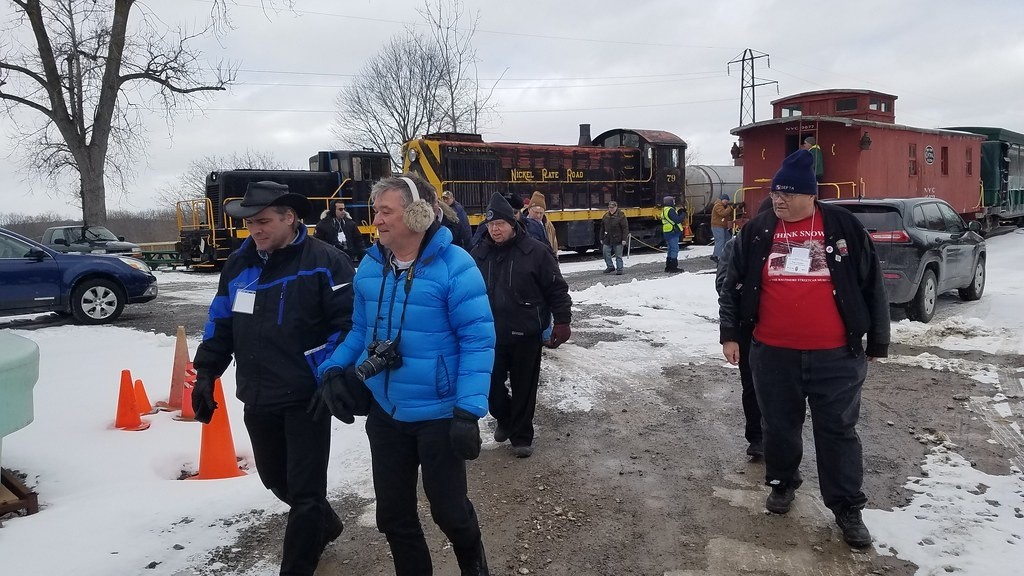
[{"x1": 32, "y1": 226, "x2": 142, "y2": 259}]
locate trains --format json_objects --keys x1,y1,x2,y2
[
  {"x1": 173, "y1": 124, "x2": 743, "y2": 257},
  {"x1": 731, "y1": 88, "x2": 1024, "y2": 237}
]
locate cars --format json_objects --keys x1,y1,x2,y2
[{"x1": 0, "y1": 226, "x2": 159, "y2": 325}]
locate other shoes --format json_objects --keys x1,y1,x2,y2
[
  {"x1": 617, "y1": 270, "x2": 622, "y2": 275},
  {"x1": 514, "y1": 445, "x2": 533, "y2": 457},
  {"x1": 665, "y1": 266, "x2": 684, "y2": 273},
  {"x1": 319, "y1": 512, "x2": 343, "y2": 554},
  {"x1": 603, "y1": 266, "x2": 615, "y2": 273},
  {"x1": 494, "y1": 419, "x2": 507, "y2": 442},
  {"x1": 710, "y1": 256, "x2": 719, "y2": 263}
]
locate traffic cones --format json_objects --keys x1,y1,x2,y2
[
  {"x1": 133, "y1": 379, "x2": 159, "y2": 416},
  {"x1": 159, "y1": 326, "x2": 192, "y2": 411},
  {"x1": 185, "y1": 377, "x2": 248, "y2": 479},
  {"x1": 107, "y1": 370, "x2": 151, "y2": 431},
  {"x1": 172, "y1": 362, "x2": 201, "y2": 422}
]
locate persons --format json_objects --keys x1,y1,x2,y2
[
  {"x1": 661, "y1": 196, "x2": 684, "y2": 272},
  {"x1": 438, "y1": 200, "x2": 462, "y2": 246},
  {"x1": 719, "y1": 150, "x2": 891, "y2": 547},
  {"x1": 523, "y1": 191, "x2": 558, "y2": 345},
  {"x1": 472, "y1": 192, "x2": 551, "y2": 248},
  {"x1": 715, "y1": 196, "x2": 773, "y2": 455},
  {"x1": 710, "y1": 194, "x2": 732, "y2": 263},
  {"x1": 318, "y1": 173, "x2": 489, "y2": 576},
  {"x1": 521, "y1": 198, "x2": 530, "y2": 212},
  {"x1": 469, "y1": 192, "x2": 572, "y2": 457},
  {"x1": 804, "y1": 137, "x2": 823, "y2": 182},
  {"x1": 442, "y1": 191, "x2": 471, "y2": 230},
  {"x1": 313, "y1": 200, "x2": 365, "y2": 266},
  {"x1": 599, "y1": 201, "x2": 628, "y2": 275},
  {"x1": 190, "y1": 180, "x2": 357, "y2": 576}
]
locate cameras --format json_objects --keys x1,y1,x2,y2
[{"x1": 354, "y1": 340, "x2": 402, "y2": 382}]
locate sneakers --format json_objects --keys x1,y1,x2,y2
[
  {"x1": 767, "y1": 478, "x2": 795, "y2": 512},
  {"x1": 746, "y1": 441, "x2": 764, "y2": 456},
  {"x1": 836, "y1": 509, "x2": 873, "y2": 546}
]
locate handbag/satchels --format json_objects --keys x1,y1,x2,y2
[{"x1": 673, "y1": 224, "x2": 680, "y2": 233}]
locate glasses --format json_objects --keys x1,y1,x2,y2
[
  {"x1": 768, "y1": 191, "x2": 803, "y2": 202},
  {"x1": 336, "y1": 207, "x2": 347, "y2": 212}
]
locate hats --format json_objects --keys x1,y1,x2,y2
[
  {"x1": 441, "y1": 190, "x2": 454, "y2": 199},
  {"x1": 486, "y1": 192, "x2": 516, "y2": 226},
  {"x1": 805, "y1": 137, "x2": 816, "y2": 145},
  {"x1": 225, "y1": 182, "x2": 310, "y2": 219},
  {"x1": 527, "y1": 192, "x2": 545, "y2": 210},
  {"x1": 770, "y1": 150, "x2": 817, "y2": 195},
  {"x1": 721, "y1": 194, "x2": 730, "y2": 200}
]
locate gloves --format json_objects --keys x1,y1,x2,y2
[
  {"x1": 307, "y1": 388, "x2": 331, "y2": 423},
  {"x1": 322, "y1": 370, "x2": 355, "y2": 424},
  {"x1": 548, "y1": 324, "x2": 570, "y2": 348},
  {"x1": 192, "y1": 369, "x2": 219, "y2": 423},
  {"x1": 447, "y1": 407, "x2": 480, "y2": 459}
]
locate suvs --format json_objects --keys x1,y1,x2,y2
[{"x1": 820, "y1": 197, "x2": 988, "y2": 323}]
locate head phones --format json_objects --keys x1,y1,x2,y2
[
  {"x1": 397, "y1": 176, "x2": 435, "y2": 232},
  {"x1": 329, "y1": 199, "x2": 338, "y2": 214}
]
